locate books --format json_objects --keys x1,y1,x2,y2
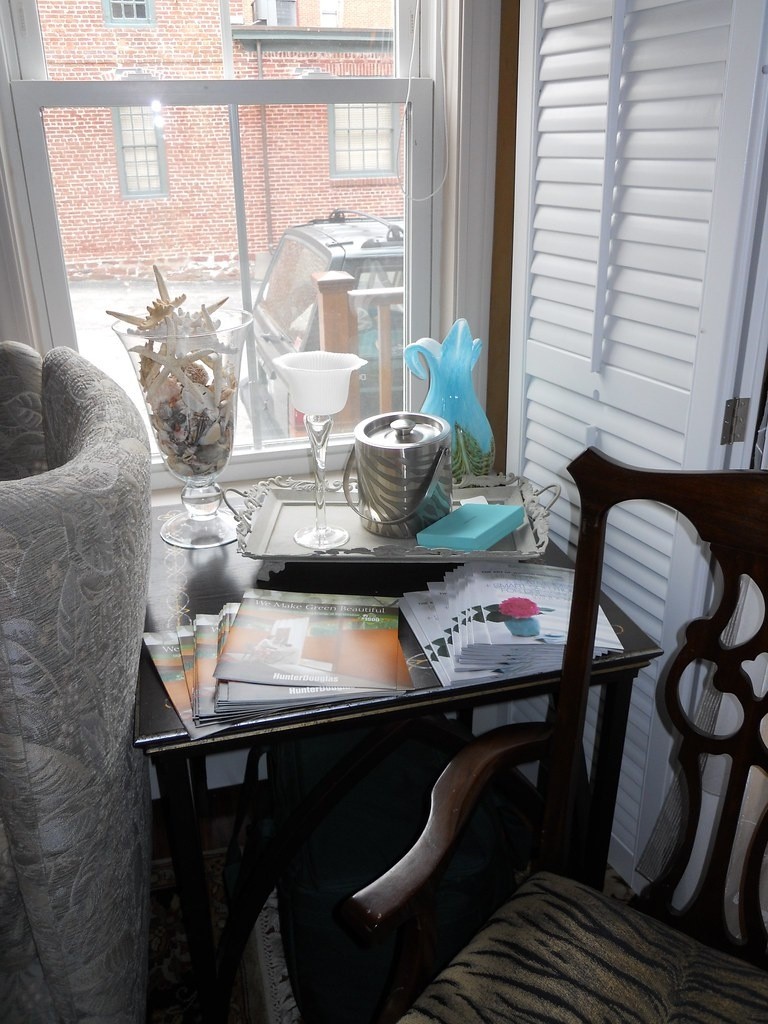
[
  {"x1": 399, "y1": 560, "x2": 625, "y2": 688},
  {"x1": 141, "y1": 588, "x2": 417, "y2": 740}
]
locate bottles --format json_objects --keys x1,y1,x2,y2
[{"x1": 403, "y1": 317, "x2": 496, "y2": 484}]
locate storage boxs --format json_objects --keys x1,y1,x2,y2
[{"x1": 418, "y1": 503, "x2": 524, "y2": 550}]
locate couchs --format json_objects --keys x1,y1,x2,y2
[{"x1": 0, "y1": 339, "x2": 152, "y2": 1024}]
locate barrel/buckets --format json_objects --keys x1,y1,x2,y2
[{"x1": 343, "y1": 410, "x2": 455, "y2": 538}]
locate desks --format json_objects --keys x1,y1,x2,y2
[{"x1": 127, "y1": 501, "x2": 666, "y2": 1024}]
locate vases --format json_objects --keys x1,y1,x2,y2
[
  {"x1": 109, "y1": 307, "x2": 254, "y2": 546},
  {"x1": 406, "y1": 316, "x2": 496, "y2": 484}
]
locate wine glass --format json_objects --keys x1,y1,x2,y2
[
  {"x1": 113, "y1": 303, "x2": 248, "y2": 551},
  {"x1": 271, "y1": 350, "x2": 368, "y2": 545}
]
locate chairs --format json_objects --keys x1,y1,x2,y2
[{"x1": 347, "y1": 444, "x2": 768, "y2": 1024}]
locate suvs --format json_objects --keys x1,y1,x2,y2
[{"x1": 238, "y1": 209, "x2": 405, "y2": 441}]
